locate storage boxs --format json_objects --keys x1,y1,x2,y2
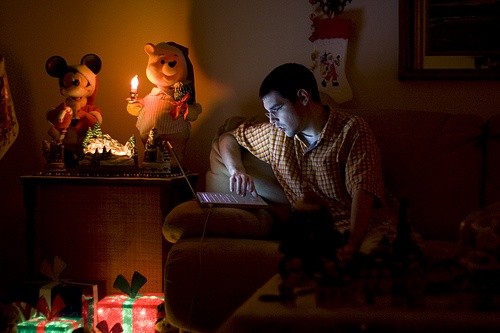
[{"x1": 17, "y1": 280, "x2": 165, "y2": 333}]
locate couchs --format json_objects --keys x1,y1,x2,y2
[{"x1": 164, "y1": 116, "x2": 500, "y2": 333}]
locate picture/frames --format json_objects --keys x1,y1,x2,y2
[{"x1": 397, "y1": 0, "x2": 500, "y2": 82}]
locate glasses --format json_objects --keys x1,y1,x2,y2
[{"x1": 265, "y1": 95, "x2": 296, "y2": 118}]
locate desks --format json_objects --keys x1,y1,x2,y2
[{"x1": 17, "y1": 174, "x2": 199, "y2": 301}]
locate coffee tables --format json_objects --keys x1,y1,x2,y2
[{"x1": 218, "y1": 273, "x2": 499, "y2": 333}]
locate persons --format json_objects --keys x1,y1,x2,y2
[{"x1": 217, "y1": 64, "x2": 439, "y2": 287}]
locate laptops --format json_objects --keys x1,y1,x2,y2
[{"x1": 167, "y1": 141, "x2": 269, "y2": 208}]
